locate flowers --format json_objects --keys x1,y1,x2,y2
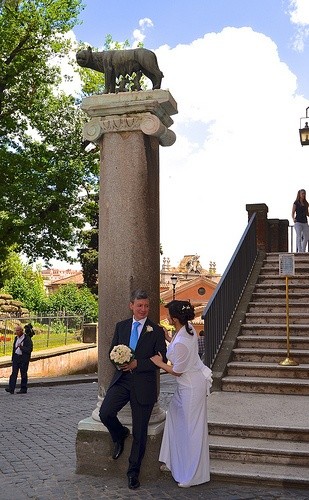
[
  {"x1": 110, "y1": 344, "x2": 133, "y2": 365},
  {"x1": 144, "y1": 325, "x2": 153, "y2": 334}
]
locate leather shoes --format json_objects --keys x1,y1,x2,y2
[
  {"x1": 112, "y1": 426, "x2": 130, "y2": 460},
  {"x1": 128, "y1": 471, "x2": 140, "y2": 489}
]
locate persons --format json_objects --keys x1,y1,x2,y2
[
  {"x1": 198, "y1": 330, "x2": 205, "y2": 358},
  {"x1": 150, "y1": 300, "x2": 213, "y2": 488},
  {"x1": 292, "y1": 189, "x2": 309, "y2": 252},
  {"x1": 99, "y1": 290, "x2": 167, "y2": 488},
  {"x1": 5, "y1": 326, "x2": 33, "y2": 394}
]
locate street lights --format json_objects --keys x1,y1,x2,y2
[{"x1": 170, "y1": 273, "x2": 177, "y2": 301}]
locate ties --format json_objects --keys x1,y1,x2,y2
[{"x1": 129, "y1": 322, "x2": 140, "y2": 351}]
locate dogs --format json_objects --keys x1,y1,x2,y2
[{"x1": 75, "y1": 45, "x2": 165, "y2": 95}]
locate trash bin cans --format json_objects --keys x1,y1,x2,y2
[
  {"x1": 268, "y1": 219, "x2": 289, "y2": 252},
  {"x1": 82, "y1": 323, "x2": 97, "y2": 343}
]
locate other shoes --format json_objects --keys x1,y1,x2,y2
[
  {"x1": 160, "y1": 464, "x2": 171, "y2": 472},
  {"x1": 178, "y1": 482, "x2": 190, "y2": 488},
  {"x1": 16, "y1": 390, "x2": 27, "y2": 394},
  {"x1": 5, "y1": 388, "x2": 14, "y2": 394}
]
